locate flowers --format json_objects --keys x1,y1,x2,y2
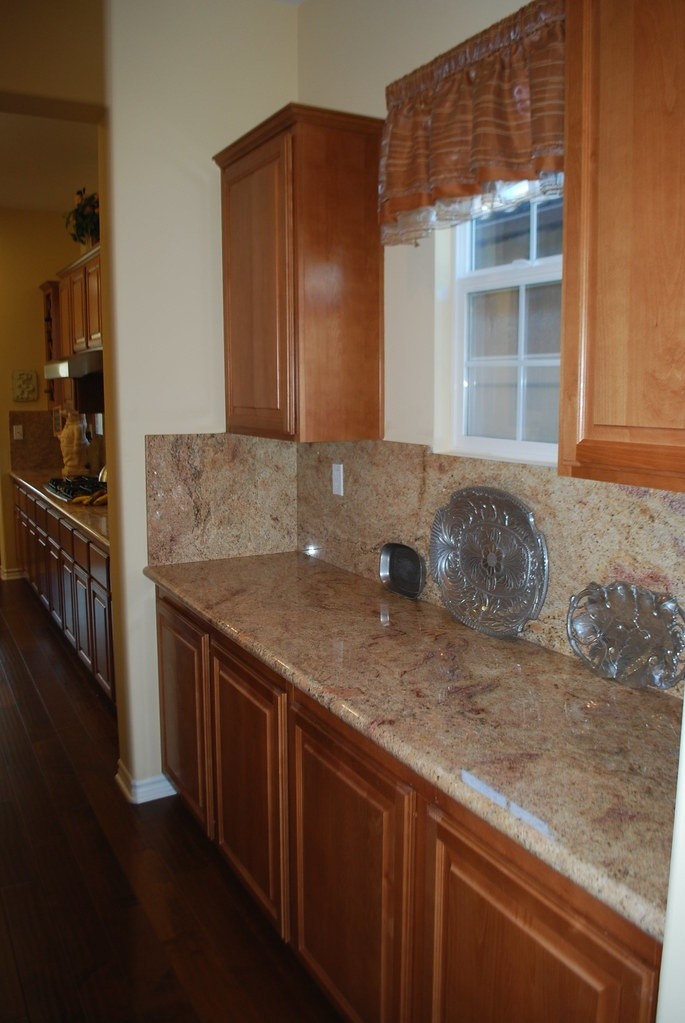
[{"x1": 64, "y1": 188, "x2": 100, "y2": 242}]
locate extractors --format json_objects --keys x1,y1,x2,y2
[{"x1": 43, "y1": 349, "x2": 104, "y2": 379}]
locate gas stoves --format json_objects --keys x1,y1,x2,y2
[{"x1": 42, "y1": 475, "x2": 107, "y2": 503}]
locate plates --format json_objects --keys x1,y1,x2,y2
[{"x1": 428, "y1": 485, "x2": 549, "y2": 639}]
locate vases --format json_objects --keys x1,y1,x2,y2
[{"x1": 79, "y1": 234, "x2": 95, "y2": 256}]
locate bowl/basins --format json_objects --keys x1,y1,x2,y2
[{"x1": 379, "y1": 542, "x2": 426, "y2": 600}]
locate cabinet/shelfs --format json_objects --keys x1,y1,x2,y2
[
  {"x1": 39, "y1": 243, "x2": 105, "y2": 414},
  {"x1": 212, "y1": 102, "x2": 384, "y2": 442},
  {"x1": 556, "y1": 0, "x2": 684, "y2": 492},
  {"x1": 10, "y1": 479, "x2": 115, "y2": 703},
  {"x1": 154, "y1": 585, "x2": 662, "y2": 1023}
]
